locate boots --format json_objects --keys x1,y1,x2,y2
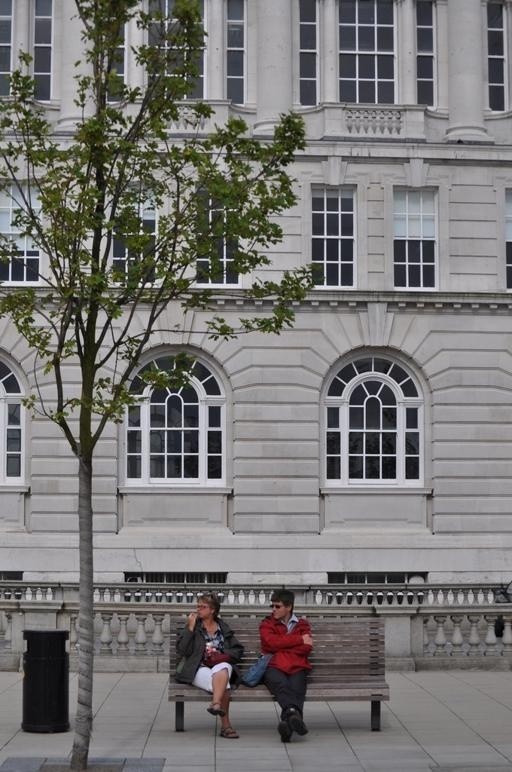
[{"x1": 277, "y1": 703, "x2": 308, "y2": 742}]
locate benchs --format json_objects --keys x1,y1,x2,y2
[{"x1": 168, "y1": 616, "x2": 390, "y2": 731}]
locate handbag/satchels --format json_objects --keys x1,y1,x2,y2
[
  {"x1": 203, "y1": 654, "x2": 230, "y2": 667},
  {"x1": 241, "y1": 654, "x2": 273, "y2": 687}
]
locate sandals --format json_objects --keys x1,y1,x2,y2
[
  {"x1": 220, "y1": 727, "x2": 239, "y2": 738},
  {"x1": 207, "y1": 703, "x2": 225, "y2": 717}
]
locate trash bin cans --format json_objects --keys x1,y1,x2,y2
[{"x1": 21, "y1": 629, "x2": 70, "y2": 732}]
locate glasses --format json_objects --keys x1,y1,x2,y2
[
  {"x1": 197, "y1": 605, "x2": 215, "y2": 609},
  {"x1": 270, "y1": 605, "x2": 280, "y2": 609}
]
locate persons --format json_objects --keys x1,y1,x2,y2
[
  {"x1": 175, "y1": 592, "x2": 245, "y2": 739},
  {"x1": 260, "y1": 590, "x2": 314, "y2": 743}
]
